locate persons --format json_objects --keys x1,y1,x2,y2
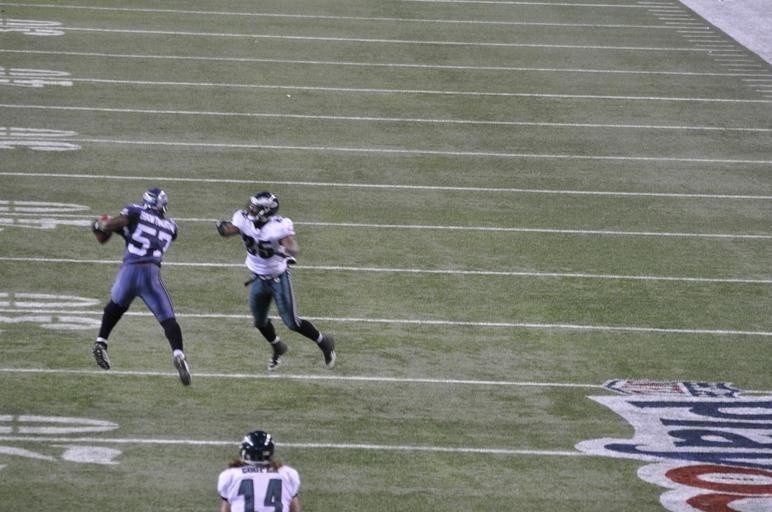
[
  {"x1": 216, "y1": 191, "x2": 336, "y2": 371},
  {"x1": 90, "y1": 187, "x2": 192, "y2": 385},
  {"x1": 217, "y1": 430, "x2": 302, "y2": 512}
]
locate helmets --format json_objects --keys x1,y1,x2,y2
[
  {"x1": 141, "y1": 187, "x2": 169, "y2": 216},
  {"x1": 247, "y1": 191, "x2": 280, "y2": 220},
  {"x1": 237, "y1": 430, "x2": 275, "y2": 467}
]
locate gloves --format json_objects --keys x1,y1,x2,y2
[{"x1": 216, "y1": 218, "x2": 234, "y2": 237}]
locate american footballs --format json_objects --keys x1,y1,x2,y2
[{"x1": 95, "y1": 214, "x2": 112, "y2": 246}]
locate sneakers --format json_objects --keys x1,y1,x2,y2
[
  {"x1": 93, "y1": 340, "x2": 111, "y2": 370},
  {"x1": 268, "y1": 343, "x2": 290, "y2": 372},
  {"x1": 317, "y1": 335, "x2": 336, "y2": 369},
  {"x1": 172, "y1": 354, "x2": 192, "y2": 385}
]
